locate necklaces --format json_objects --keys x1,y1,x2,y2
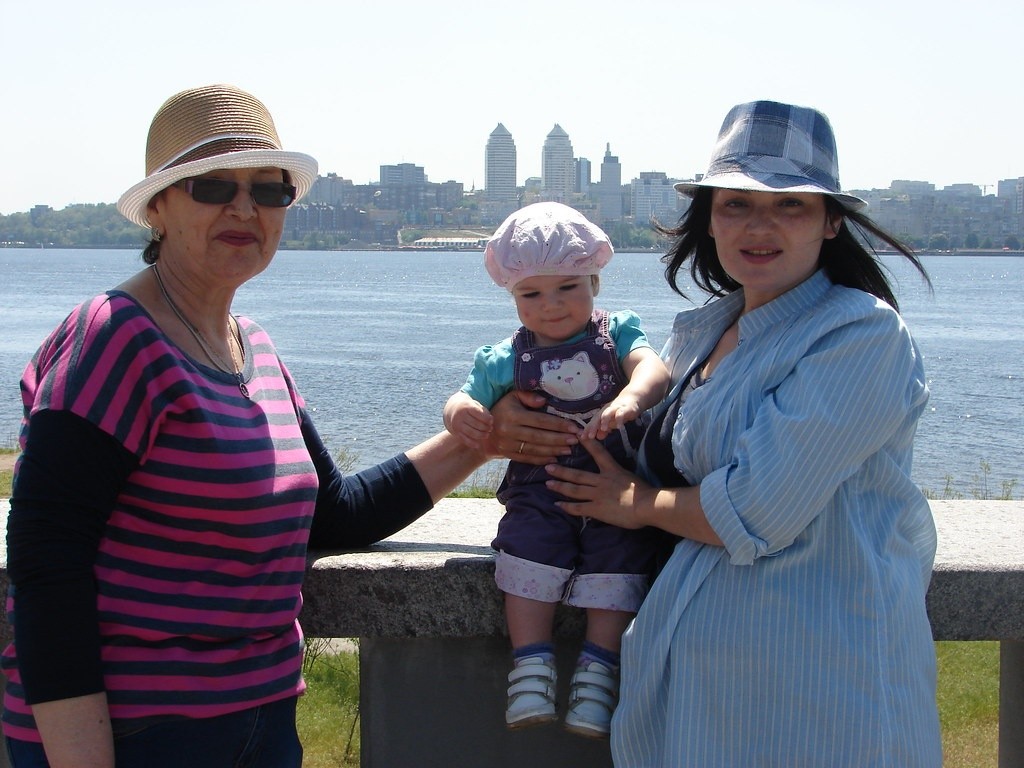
[{"x1": 154, "y1": 263, "x2": 250, "y2": 398}]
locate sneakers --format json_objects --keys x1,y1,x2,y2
[
  {"x1": 505, "y1": 652, "x2": 563, "y2": 731},
  {"x1": 564, "y1": 662, "x2": 616, "y2": 739}
]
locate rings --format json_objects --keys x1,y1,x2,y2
[{"x1": 517, "y1": 441, "x2": 525, "y2": 454}]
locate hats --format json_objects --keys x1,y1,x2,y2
[
  {"x1": 482, "y1": 201, "x2": 614, "y2": 295},
  {"x1": 672, "y1": 101, "x2": 867, "y2": 212},
  {"x1": 116, "y1": 84, "x2": 319, "y2": 229}
]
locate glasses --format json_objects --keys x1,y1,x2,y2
[{"x1": 171, "y1": 178, "x2": 297, "y2": 208}]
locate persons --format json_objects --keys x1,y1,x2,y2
[
  {"x1": 441, "y1": 199, "x2": 670, "y2": 742},
  {"x1": 486, "y1": 100, "x2": 945, "y2": 766},
  {"x1": 5, "y1": 87, "x2": 488, "y2": 767}
]
